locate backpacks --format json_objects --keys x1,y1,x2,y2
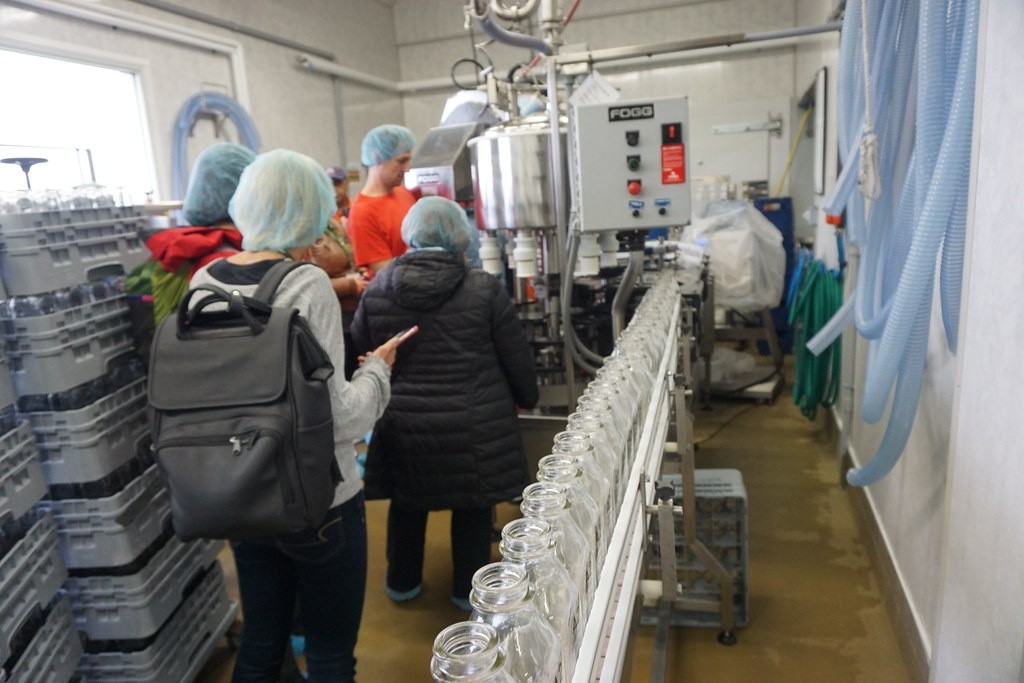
[{"x1": 142, "y1": 260, "x2": 346, "y2": 542}]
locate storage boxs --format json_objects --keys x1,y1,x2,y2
[
  {"x1": 73, "y1": 560, "x2": 243, "y2": 683},
  {"x1": 0, "y1": 415, "x2": 51, "y2": 559},
  {"x1": 636, "y1": 467, "x2": 750, "y2": 628},
  {"x1": 38, "y1": 463, "x2": 183, "y2": 576},
  {"x1": 5, "y1": 578, "x2": 88, "y2": 683},
  {"x1": 0, "y1": 197, "x2": 152, "y2": 317},
  {"x1": 69, "y1": 532, "x2": 226, "y2": 641},
  {"x1": 0, "y1": 505, "x2": 71, "y2": 682},
  {"x1": 22, "y1": 372, "x2": 154, "y2": 501},
  {"x1": 0, "y1": 293, "x2": 150, "y2": 412}
]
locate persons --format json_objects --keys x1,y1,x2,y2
[
  {"x1": 188, "y1": 148, "x2": 397, "y2": 683},
  {"x1": 135, "y1": 142, "x2": 312, "y2": 650},
  {"x1": 347, "y1": 124, "x2": 418, "y2": 275},
  {"x1": 348, "y1": 194, "x2": 540, "y2": 608}
]
